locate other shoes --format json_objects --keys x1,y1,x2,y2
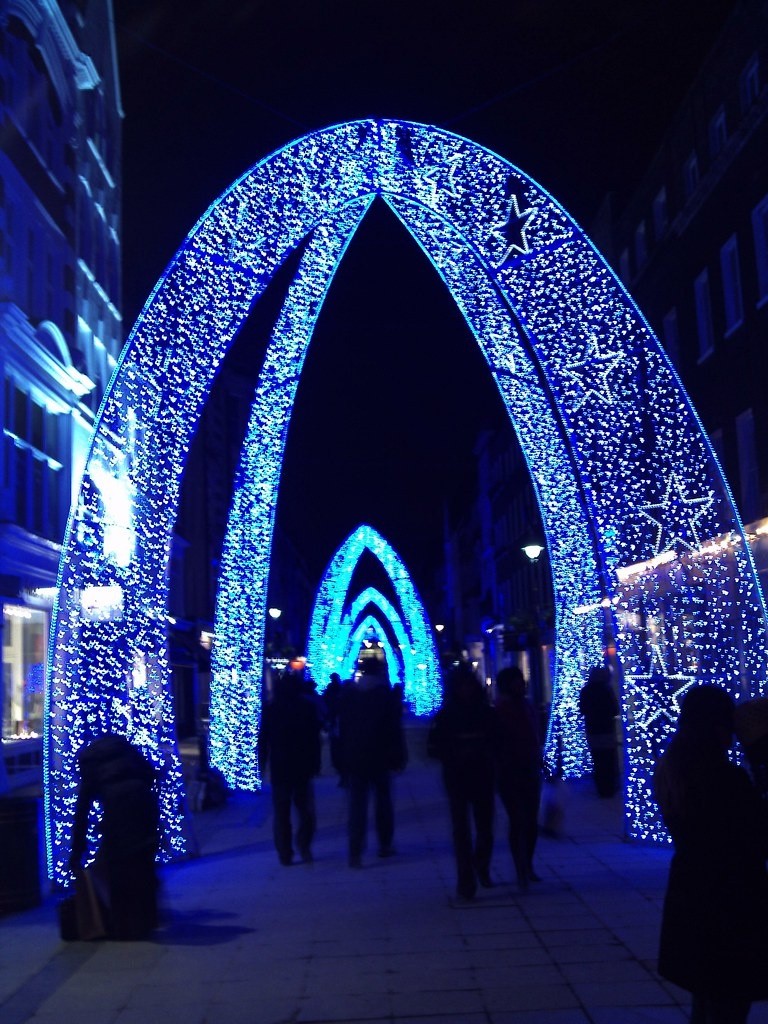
[
  {"x1": 298, "y1": 840, "x2": 313, "y2": 862},
  {"x1": 377, "y1": 847, "x2": 399, "y2": 858}
]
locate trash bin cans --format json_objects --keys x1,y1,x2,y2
[{"x1": 0, "y1": 787, "x2": 44, "y2": 913}]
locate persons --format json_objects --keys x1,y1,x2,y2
[
  {"x1": 58, "y1": 735, "x2": 163, "y2": 942},
  {"x1": 580, "y1": 667, "x2": 620, "y2": 798},
  {"x1": 427, "y1": 660, "x2": 543, "y2": 900},
  {"x1": 658, "y1": 685, "x2": 768, "y2": 1024},
  {"x1": 259, "y1": 657, "x2": 402, "y2": 869}
]
